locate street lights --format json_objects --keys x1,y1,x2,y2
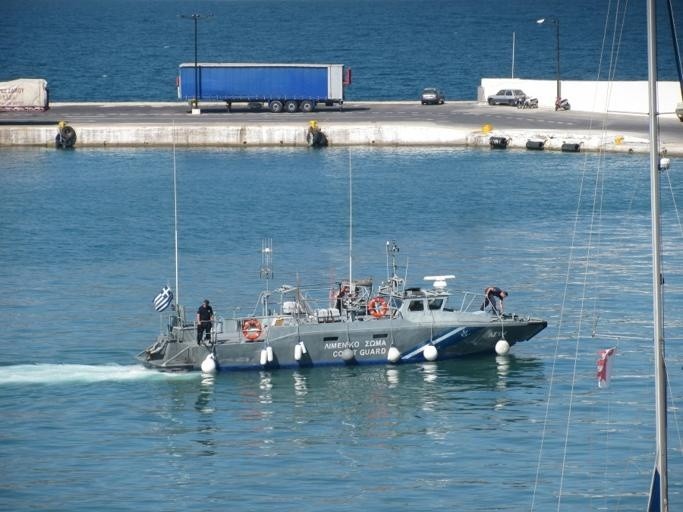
[
  {"x1": 536, "y1": 14, "x2": 560, "y2": 110},
  {"x1": 177, "y1": 11, "x2": 215, "y2": 109}
]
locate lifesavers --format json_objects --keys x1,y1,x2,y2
[
  {"x1": 369, "y1": 298, "x2": 387, "y2": 317},
  {"x1": 243, "y1": 320, "x2": 261, "y2": 339}
]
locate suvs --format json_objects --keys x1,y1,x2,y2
[
  {"x1": 420, "y1": 88, "x2": 445, "y2": 105},
  {"x1": 487, "y1": 89, "x2": 528, "y2": 107}
]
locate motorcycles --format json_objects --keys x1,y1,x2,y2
[
  {"x1": 517, "y1": 97, "x2": 538, "y2": 109},
  {"x1": 554, "y1": 97, "x2": 571, "y2": 110}
]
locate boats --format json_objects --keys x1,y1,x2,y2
[{"x1": 134, "y1": 116, "x2": 548, "y2": 375}]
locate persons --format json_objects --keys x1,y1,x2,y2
[
  {"x1": 479, "y1": 287, "x2": 506, "y2": 314},
  {"x1": 195, "y1": 300, "x2": 212, "y2": 345}
]
